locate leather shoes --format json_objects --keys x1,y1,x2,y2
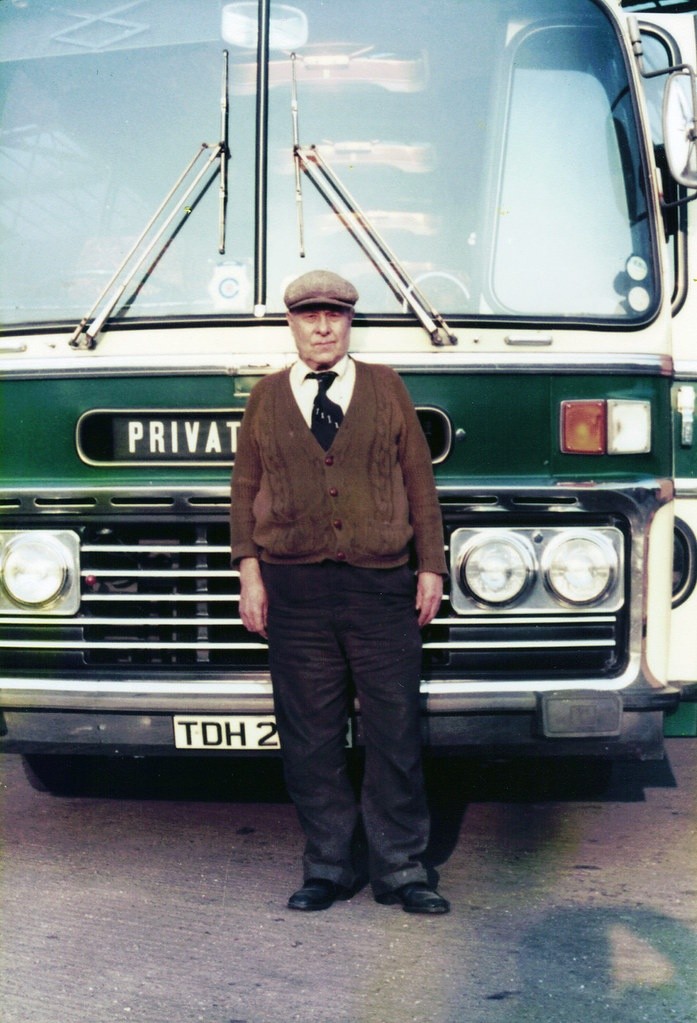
[
  {"x1": 374, "y1": 881, "x2": 451, "y2": 913},
  {"x1": 288, "y1": 877, "x2": 354, "y2": 911}
]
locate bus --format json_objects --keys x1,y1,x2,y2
[{"x1": 0, "y1": 1, "x2": 697, "y2": 799}]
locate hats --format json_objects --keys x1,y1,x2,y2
[{"x1": 284, "y1": 270, "x2": 359, "y2": 311}]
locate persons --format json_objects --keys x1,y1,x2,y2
[{"x1": 231, "y1": 270, "x2": 450, "y2": 913}]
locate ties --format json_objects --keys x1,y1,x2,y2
[{"x1": 306, "y1": 372, "x2": 343, "y2": 451}]
